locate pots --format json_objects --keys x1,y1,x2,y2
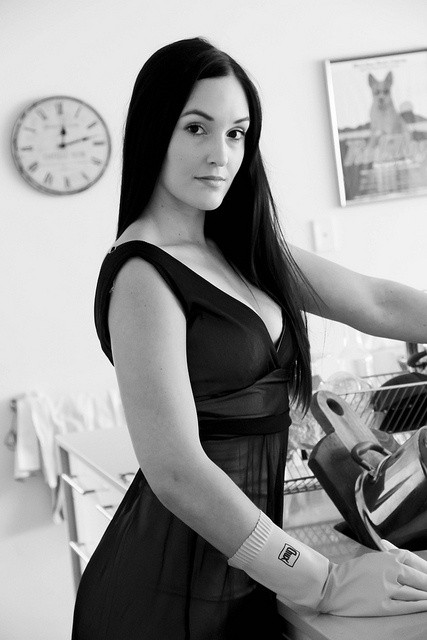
[{"x1": 352, "y1": 425, "x2": 427, "y2": 551}]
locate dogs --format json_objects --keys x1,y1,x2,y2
[{"x1": 365, "y1": 71, "x2": 409, "y2": 152}]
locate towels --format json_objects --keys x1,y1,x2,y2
[{"x1": 11, "y1": 384, "x2": 129, "y2": 493}]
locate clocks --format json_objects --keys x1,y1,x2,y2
[{"x1": 10, "y1": 94, "x2": 112, "y2": 196}]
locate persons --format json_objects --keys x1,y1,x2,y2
[{"x1": 71, "y1": 35, "x2": 426, "y2": 634}]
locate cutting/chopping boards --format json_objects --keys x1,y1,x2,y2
[{"x1": 308, "y1": 389, "x2": 404, "y2": 547}]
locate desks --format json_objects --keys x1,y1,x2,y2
[{"x1": 54, "y1": 424, "x2": 426, "y2": 639}]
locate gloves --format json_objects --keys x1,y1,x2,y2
[{"x1": 228, "y1": 509, "x2": 427, "y2": 616}]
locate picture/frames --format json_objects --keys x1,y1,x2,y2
[{"x1": 323, "y1": 45, "x2": 426, "y2": 208}]
